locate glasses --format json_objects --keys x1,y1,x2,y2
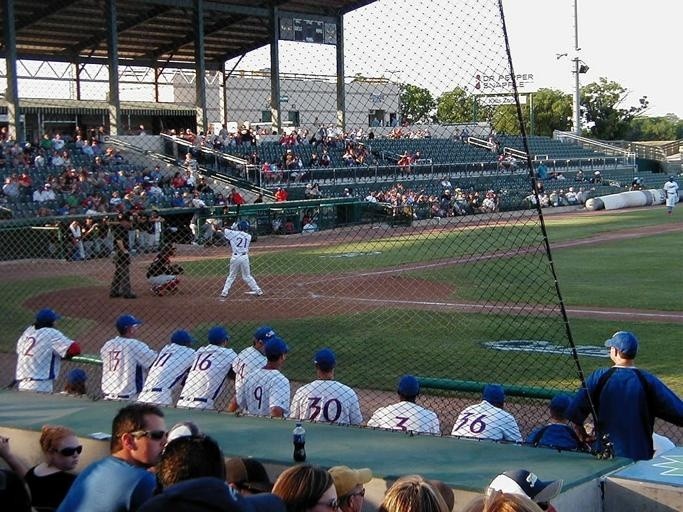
[
  {"x1": 346, "y1": 488, "x2": 366, "y2": 498},
  {"x1": 237, "y1": 485, "x2": 260, "y2": 495},
  {"x1": 314, "y1": 498, "x2": 342, "y2": 509},
  {"x1": 128, "y1": 430, "x2": 168, "y2": 442},
  {"x1": 49, "y1": 444, "x2": 85, "y2": 458}
]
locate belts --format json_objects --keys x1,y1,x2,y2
[
  {"x1": 15, "y1": 377, "x2": 51, "y2": 384},
  {"x1": 141, "y1": 386, "x2": 168, "y2": 396},
  {"x1": 102, "y1": 391, "x2": 132, "y2": 401},
  {"x1": 179, "y1": 396, "x2": 211, "y2": 404},
  {"x1": 232, "y1": 252, "x2": 247, "y2": 255}
]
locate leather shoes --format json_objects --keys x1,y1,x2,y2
[
  {"x1": 245, "y1": 289, "x2": 264, "y2": 297},
  {"x1": 124, "y1": 292, "x2": 137, "y2": 299},
  {"x1": 109, "y1": 292, "x2": 123, "y2": 298}
]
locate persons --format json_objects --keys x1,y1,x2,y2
[
  {"x1": 0, "y1": 403, "x2": 564, "y2": 511},
  {"x1": 15, "y1": 310, "x2": 81, "y2": 392},
  {"x1": 60, "y1": 367, "x2": 89, "y2": 400},
  {"x1": 563, "y1": 330, "x2": 682, "y2": 462},
  {"x1": 526, "y1": 393, "x2": 580, "y2": 449},
  {"x1": 366, "y1": 375, "x2": 439, "y2": 433},
  {"x1": 137, "y1": 329, "x2": 196, "y2": 407},
  {"x1": 213, "y1": 221, "x2": 263, "y2": 298},
  {"x1": 175, "y1": 327, "x2": 237, "y2": 409},
  {"x1": 228, "y1": 338, "x2": 290, "y2": 417},
  {"x1": 0, "y1": 126, "x2": 680, "y2": 258},
  {"x1": 229, "y1": 326, "x2": 277, "y2": 392},
  {"x1": 99, "y1": 314, "x2": 157, "y2": 402},
  {"x1": 451, "y1": 383, "x2": 523, "y2": 441},
  {"x1": 289, "y1": 348, "x2": 363, "y2": 424},
  {"x1": 110, "y1": 212, "x2": 136, "y2": 299},
  {"x1": 145, "y1": 235, "x2": 180, "y2": 297}
]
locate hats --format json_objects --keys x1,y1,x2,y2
[
  {"x1": 314, "y1": 348, "x2": 335, "y2": 366},
  {"x1": 224, "y1": 455, "x2": 275, "y2": 494},
  {"x1": 329, "y1": 465, "x2": 373, "y2": 497},
  {"x1": 116, "y1": 314, "x2": 143, "y2": 329},
  {"x1": 549, "y1": 392, "x2": 573, "y2": 413},
  {"x1": 397, "y1": 375, "x2": 419, "y2": 396},
  {"x1": 487, "y1": 467, "x2": 565, "y2": 504},
  {"x1": 482, "y1": 383, "x2": 507, "y2": 404},
  {"x1": 67, "y1": 368, "x2": 88, "y2": 383},
  {"x1": 208, "y1": 327, "x2": 231, "y2": 345},
  {"x1": 165, "y1": 420, "x2": 206, "y2": 454},
  {"x1": 253, "y1": 326, "x2": 280, "y2": 344},
  {"x1": 37, "y1": 309, "x2": 60, "y2": 321},
  {"x1": 265, "y1": 336, "x2": 290, "y2": 356},
  {"x1": 604, "y1": 330, "x2": 639, "y2": 357},
  {"x1": 171, "y1": 330, "x2": 195, "y2": 345},
  {"x1": 119, "y1": 214, "x2": 132, "y2": 223}
]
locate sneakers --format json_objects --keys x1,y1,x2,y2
[
  {"x1": 220, "y1": 292, "x2": 227, "y2": 298},
  {"x1": 150, "y1": 286, "x2": 163, "y2": 297}
]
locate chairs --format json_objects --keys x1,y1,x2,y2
[{"x1": 0, "y1": 136, "x2": 683, "y2": 218}]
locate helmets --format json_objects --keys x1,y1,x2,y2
[{"x1": 237, "y1": 221, "x2": 247, "y2": 232}]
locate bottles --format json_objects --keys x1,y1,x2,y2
[{"x1": 292, "y1": 422, "x2": 307, "y2": 464}]
